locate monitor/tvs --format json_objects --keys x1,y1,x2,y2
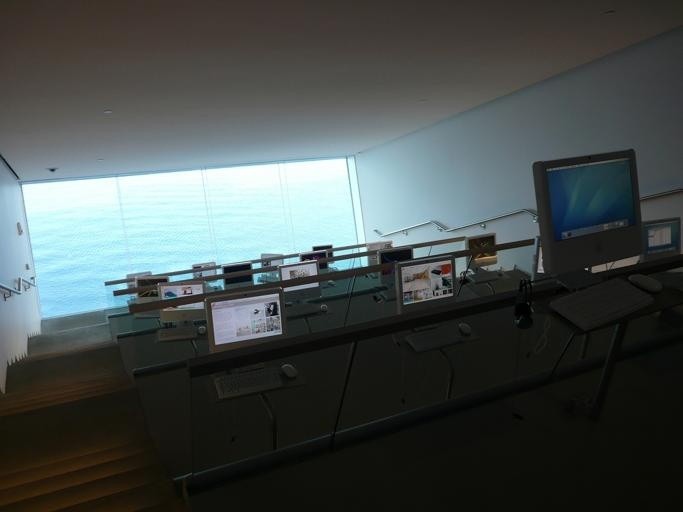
[
  {"x1": 205, "y1": 287, "x2": 287, "y2": 351},
  {"x1": 643, "y1": 217, "x2": 681, "y2": 263},
  {"x1": 377, "y1": 246, "x2": 413, "y2": 275},
  {"x1": 395, "y1": 255, "x2": 457, "y2": 313},
  {"x1": 158, "y1": 282, "x2": 206, "y2": 322},
  {"x1": 135, "y1": 276, "x2": 169, "y2": 297},
  {"x1": 222, "y1": 262, "x2": 253, "y2": 285},
  {"x1": 312, "y1": 245, "x2": 334, "y2": 264},
  {"x1": 261, "y1": 253, "x2": 284, "y2": 271},
  {"x1": 533, "y1": 149, "x2": 648, "y2": 272},
  {"x1": 299, "y1": 250, "x2": 328, "y2": 273},
  {"x1": 126, "y1": 271, "x2": 152, "y2": 287},
  {"x1": 279, "y1": 260, "x2": 322, "y2": 301},
  {"x1": 534, "y1": 236, "x2": 546, "y2": 277},
  {"x1": 465, "y1": 233, "x2": 498, "y2": 267},
  {"x1": 192, "y1": 261, "x2": 218, "y2": 283}
]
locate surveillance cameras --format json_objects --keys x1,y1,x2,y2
[{"x1": 50, "y1": 169, "x2": 55, "y2": 171}]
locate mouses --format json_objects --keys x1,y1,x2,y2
[{"x1": 280, "y1": 364, "x2": 298, "y2": 379}]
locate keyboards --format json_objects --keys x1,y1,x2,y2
[{"x1": 214, "y1": 368, "x2": 283, "y2": 400}]
